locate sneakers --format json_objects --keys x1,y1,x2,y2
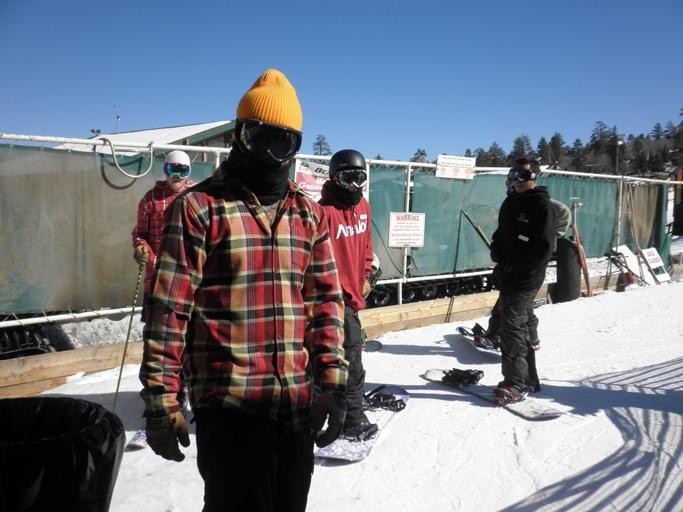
[
  {"x1": 344, "y1": 414, "x2": 378, "y2": 442},
  {"x1": 492, "y1": 382, "x2": 522, "y2": 402}
]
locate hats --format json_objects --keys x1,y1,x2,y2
[{"x1": 238, "y1": 68, "x2": 303, "y2": 132}]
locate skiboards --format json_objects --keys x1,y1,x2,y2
[{"x1": 126, "y1": 393, "x2": 188, "y2": 448}]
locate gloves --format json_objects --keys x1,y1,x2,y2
[
  {"x1": 312, "y1": 387, "x2": 347, "y2": 448},
  {"x1": 142, "y1": 409, "x2": 190, "y2": 462},
  {"x1": 133, "y1": 245, "x2": 150, "y2": 265},
  {"x1": 362, "y1": 280, "x2": 371, "y2": 299}
]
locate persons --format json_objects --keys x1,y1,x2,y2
[
  {"x1": 316, "y1": 151, "x2": 380, "y2": 441},
  {"x1": 130, "y1": 150, "x2": 194, "y2": 418},
  {"x1": 140, "y1": 69, "x2": 350, "y2": 510},
  {"x1": 472, "y1": 199, "x2": 572, "y2": 353},
  {"x1": 490, "y1": 158, "x2": 555, "y2": 402}
]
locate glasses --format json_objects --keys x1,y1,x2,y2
[
  {"x1": 335, "y1": 169, "x2": 367, "y2": 189},
  {"x1": 164, "y1": 162, "x2": 192, "y2": 179},
  {"x1": 238, "y1": 118, "x2": 302, "y2": 164},
  {"x1": 508, "y1": 167, "x2": 534, "y2": 182}
]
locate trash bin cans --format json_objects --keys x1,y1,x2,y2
[{"x1": 0, "y1": 395, "x2": 127, "y2": 512}]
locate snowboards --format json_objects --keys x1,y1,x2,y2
[
  {"x1": 424, "y1": 367, "x2": 561, "y2": 422},
  {"x1": 457, "y1": 323, "x2": 503, "y2": 358},
  {"x1": 313, "y1": 384, "x2": 410, "y2": 462}
]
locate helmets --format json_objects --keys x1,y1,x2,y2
[
  {"x1": 164, "y1": 150, "x2": 190, "y2": 166},
  {"x1": 329, "y1": 149, "x2": 368, "y2": 179}
]
